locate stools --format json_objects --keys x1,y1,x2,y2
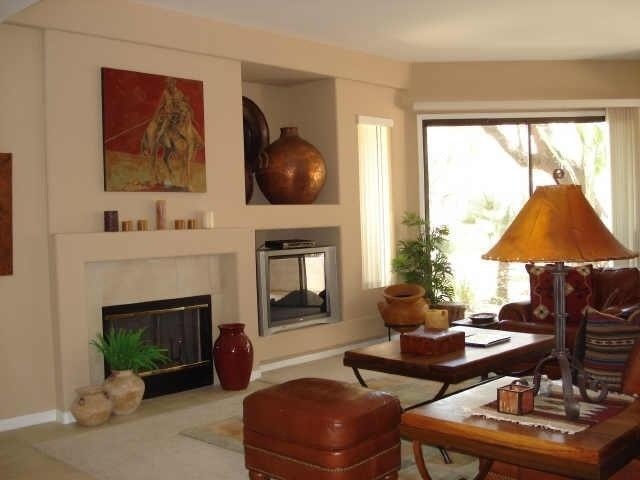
[{"x1": 244, "y1": 379, "x2": 400, "y2": 479}]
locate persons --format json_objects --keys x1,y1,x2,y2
[{"x1": 152, "y1": 77, "x2": 191, "y2": 148}]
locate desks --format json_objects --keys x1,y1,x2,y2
[
  {"x1": 344, "y1": 326, "x2": 558, "y2": 465},
  {"x1": 400, "y1": 375, "x2": 639, "y2": 479}
]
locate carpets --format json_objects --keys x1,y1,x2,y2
[{"x1": 181, "y1": 368, "x2": 498, "y2": 480}]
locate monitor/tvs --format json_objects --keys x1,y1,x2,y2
[{"x1": 256, "y1": 245, "x2": 341, "y2": 337}]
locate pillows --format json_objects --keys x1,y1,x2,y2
[
  {"x1": 523, "y1": 260, "x2": 594, "y2": 326},
  {"x1": 579, "y1": 304, "x2": 639, "y2": 393}
]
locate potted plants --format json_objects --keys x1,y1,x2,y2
[
  {"x1": 388, "y1": 210, "x2": 458, "y2": 335},
  {"x1": 88, "y1": 324, "x2": 177, "y2": 416}
]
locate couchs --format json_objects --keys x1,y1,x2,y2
[{"x1": 496, "y1": 262, "x2": 640, "y2": 397}]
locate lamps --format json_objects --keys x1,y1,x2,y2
[{"x1": 479, "y1": 168, "x2": 640, "y2": 421}]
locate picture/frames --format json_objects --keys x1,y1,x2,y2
[
  {"x1": 100, "y1": 67, "x2": 207, "y2": 194},
  {"x1": 0, "y1": 152, "x2": 13, "y2": 276}
]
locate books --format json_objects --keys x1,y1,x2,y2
[{"x1": 465, "y1": 333, "x2": 511, "y2": 348}]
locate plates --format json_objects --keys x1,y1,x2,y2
[{"x1": 469, "y1": 312, "x2": 497, "y2": 323}]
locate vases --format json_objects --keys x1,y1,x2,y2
[
  {"x1": 377, "y1": 283, "x2": 432, "y2": 333},
  {"x1": 71, "y1": 385, "x2": 116, "y2": 428},
  {"x1": 213, "y1": 322, "x2": 255, "y2": 390}
]
